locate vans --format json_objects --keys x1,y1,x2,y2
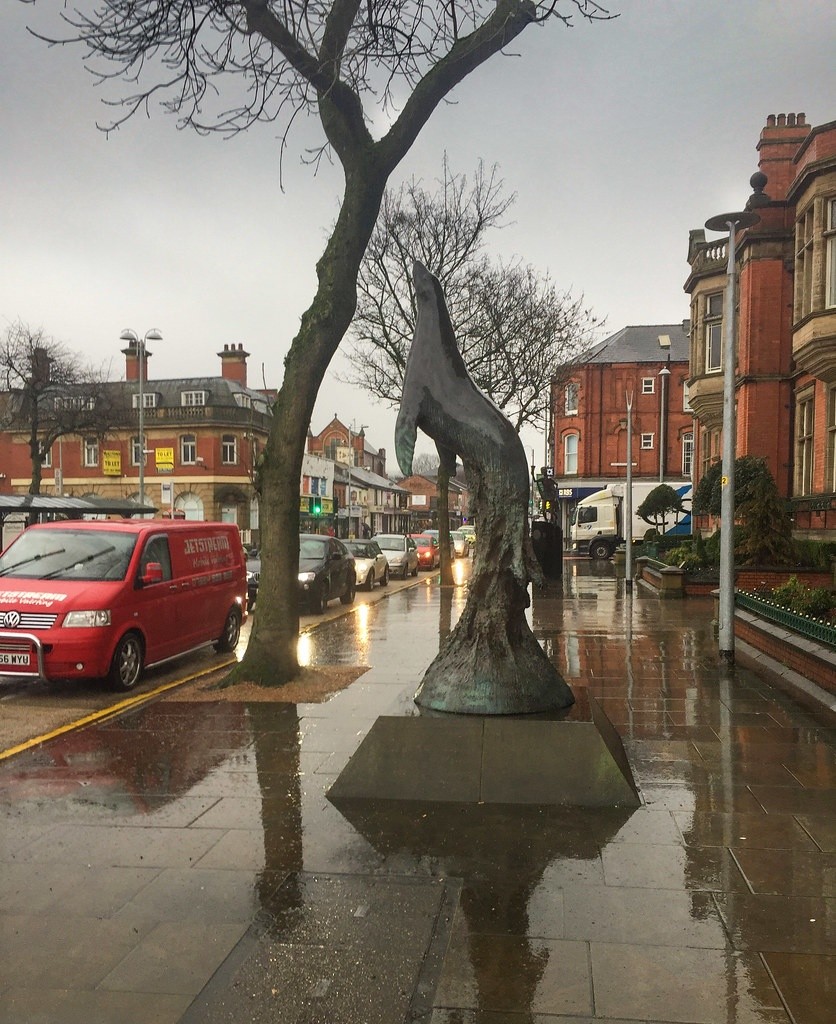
[{"x1": 0, "y1": 518, "x2": 250, "y2": 692}]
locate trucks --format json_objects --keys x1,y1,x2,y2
[{"x1": 569, "y1": 482, "x2": 693, "y2": 563}]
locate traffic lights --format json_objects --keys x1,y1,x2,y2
[
  {"x1": 313, "y1": 497, "x2": 323, "y2": 515},
  {"x1": 307, "y1": 497, "x2": 315, "y2": 516}
]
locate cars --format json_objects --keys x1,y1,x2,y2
[
  {"x1": 338, "y1": 539, "x2": 390, "y2": 591},
  {"x1": 459, "y1": 525, "x2": 477, "y2": 547},
  {"x1": 247, "y1": 534, "x2": 356, "y2": 616},
  {"x1": 370, "y1": 534, "x2": 421, "y2": 580},
  {"x1": 423, "y1": 530, "x2": 456, "y2": 564},
  {"x1": 450, "y1": 531, "x2": 470, "y2": 558},
  {"x1": 406, "y1": 533, "x2": 441, "y2": 571}
]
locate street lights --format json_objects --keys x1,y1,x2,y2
[
  {"x1": 347, "y1": 425, "x2": 370, "y2": 536},
  {"x1": 119, "y1": 327, "x2": 165, "y2": 519},
  {"x1": 705, "y1": 211, "x2": 762, "y2": 665}
]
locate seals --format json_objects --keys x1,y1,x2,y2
[{"x1": 395, "y1": 260, "x2": 550, "y2": 594}]
